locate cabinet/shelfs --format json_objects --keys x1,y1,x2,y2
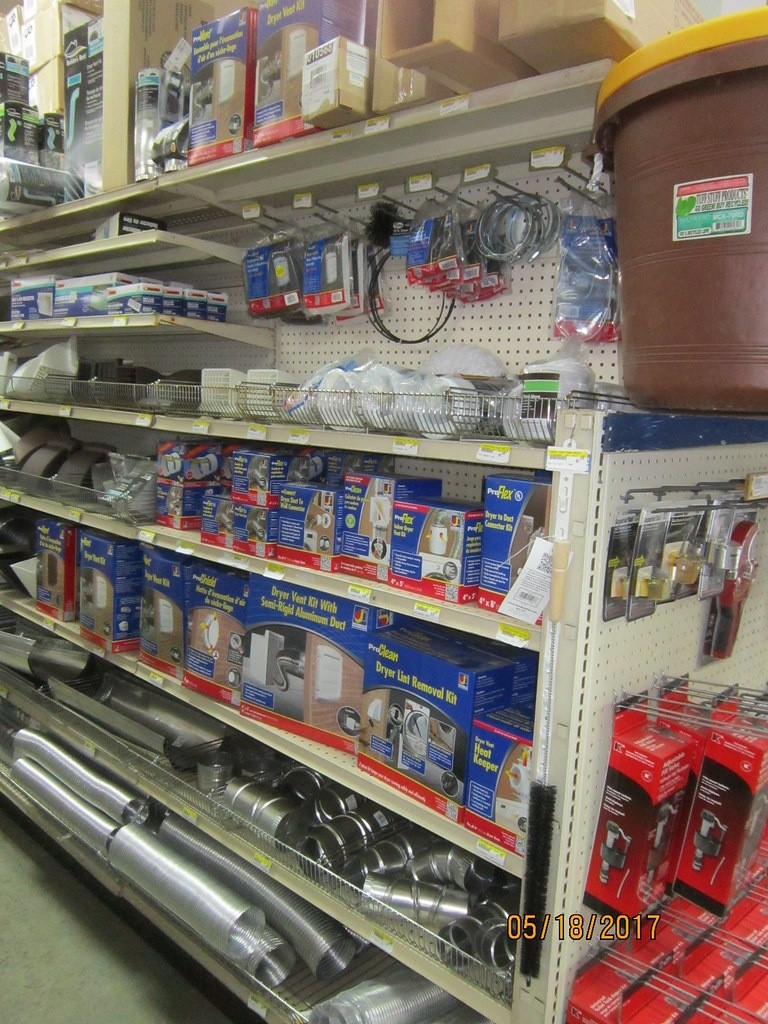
[{"x1": 0, "y1": 57, "x2": 768, "y2": 1024}]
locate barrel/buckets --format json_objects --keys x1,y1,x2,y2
[
  {"x1": 596, "y1": 7, "x2": 768, "y2": 109},
  {"x1": 592, "y1": 36, "x2": 768, "y2": 412}
]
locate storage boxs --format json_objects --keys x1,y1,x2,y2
[
  {"x1": 300, "y1": 35, "x2": 375, "y2": 130},
  {"x1": 562, "y1": 673, "x2": 768, "y2": 1024},
  {"x1": 498, "y1": 0, "x2": 705, "y2": 75},
  {"x1": 163, "y1": 287, "x2": 183, "y2": 316},
  {"x1": 183, "y1": 287, "x2": 207, "y2": 320},
  {"x1": 371, "y1": 0, "x2": 541, "y2": 117},
  {"x1": 252, "y1": 0, "x2": 378, "y2": 148},
  {"x1": 89, "y1": 210, "x2": 168, "y2": 241},
  {"x1": 33, "y1": 438, "x2": 552, "y2": 860},
  {"x1": 10, "y1": 274, "x2": 75, "y2": 319},
  {"x1": 163, "y1": 281, "x2": 194, "y2": 289},
  {"x1": 139, "y1": 277, "x2": 164, "y2": 285},
  {"x1": 206, "y1": 292, "x2": 229, "y2": 323},
  {"x1": 54, "y1": 271, "x2": 140, "y2": 317},
  {"x1": 187, "y1": 7, "x2": 259, "y2": 163},
  {"x1": 106, "y1": 283, "x2": 164, "y2": 315}
]
